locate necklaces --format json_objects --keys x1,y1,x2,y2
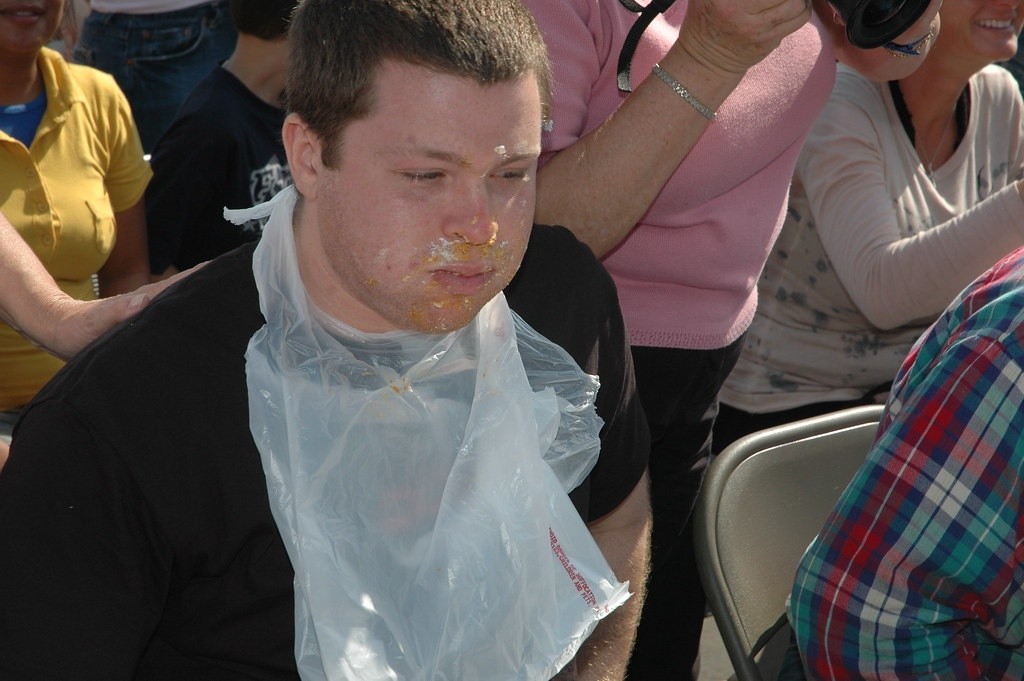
[{"x1": 914, "y1": 103, "x2": 957, "y2": 190}]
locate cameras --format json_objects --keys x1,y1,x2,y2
[{"x1": 827, "y1": 0, "x2": 931, "y2": 49}]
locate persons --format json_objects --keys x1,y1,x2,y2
[
  {"x1": 0, "y1": 0, "x2": 153, "y2": 470},
  {"x1": 145, "y1": 0, "x2": 301, "y2": 278},
  {"x1": 73, "y1": 0, "x2": 238, "y2": 157},
  {"x1": 0, "y1": 210, "x2": 211, "y2": 362},
  {"x1": 789, "y1": 246, "x2": 1024, "y2": 680},
  {"x1": 0, "y1": 0, "x2": 654, "y2": 681},
  {"x1": 523, "y1": 0, "x2": 942, "y2": 681},
  {"x1": 709, "y1": 0, "x2": 1024, "y2": 456}
]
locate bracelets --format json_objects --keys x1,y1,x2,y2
[
  {"x1": 879, "y1": 21, "x2": 934, "y2": 59},
  {"x1": 651, "y1": 64, "x2": 715, "y2": 121}
]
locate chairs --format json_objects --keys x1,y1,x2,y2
[{"x1": 697, "y1": 405, "x2": 892, "y2": 681}]
